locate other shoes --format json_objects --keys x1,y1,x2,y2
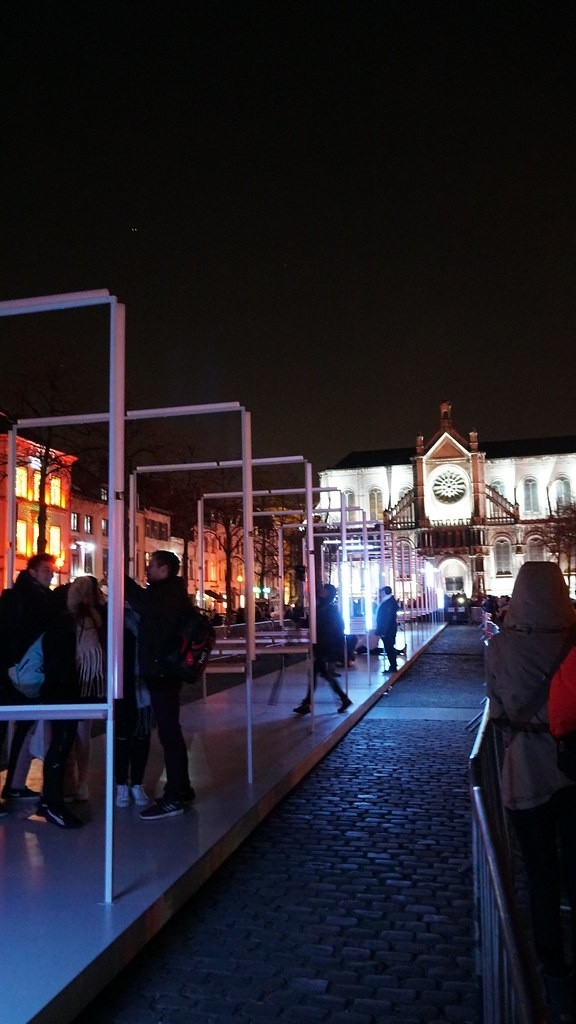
[
  {"x1": 382, "y1": 667, "x2": 397, "y2": 673},
  {"x1": 338, "y1": 695, "x2": 352, "y2": 712},
  {"x1": 293, "y1": 705, "x2": 311, "y2": 714}
]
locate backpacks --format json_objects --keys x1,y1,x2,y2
[{"x1": 169, "y1": 611, "x2": 216, "y2": 684}]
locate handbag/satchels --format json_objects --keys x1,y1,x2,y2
[{"x1": 8, "y1": 631, "x2": 46, "y2": 698}]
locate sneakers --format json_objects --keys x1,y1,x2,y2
[
  {"x1": 114, "y1": 784, "x2": 130, "y2": 808},
  {"x1": 154, "y1": 788, "x2": 196, "y2": 804},
  {"x1": 35, "y1": 798, "x2": 81, "y2": 829},
  {"x1": 139, "y1": 797, "x2": 184, "y2": 819},
  {"x1": 130, "y1": 785, "x2": 150, "y2": 806},
  {"x1": 1, "y1": 784, "x2": 41, "y2": 800},
  {"x1": 74, "y1": 782, "x2": 89, "y2": 800},
  {"x1": 63, "y1": 795, "x2": 75, "y2": 802}
]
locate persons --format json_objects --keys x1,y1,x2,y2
[
  {"x1": 123, "y1": 549, "x2": 197, "y2": 820},
  {"x1": 486, "y1": 561, "x2": 576, "y2": 1024},
  {"x1": 292, "y1": 584, "x2": 353, "y2": 714},
  {"x1": 484, "y1": 594, "x2": 576, "y2": 624},
  {"x1": 356, "y1": 630, "x2": 407, "y2": 656},
  {"x1": 0, "y1": 554, "x2": 155, "y2": 829},
  {"x1": 186, "y1": 594, "x2": 310, "y2": 628},
  {"x1": 375, "y1": 586, "x2": 398, "y2": 674}
]
